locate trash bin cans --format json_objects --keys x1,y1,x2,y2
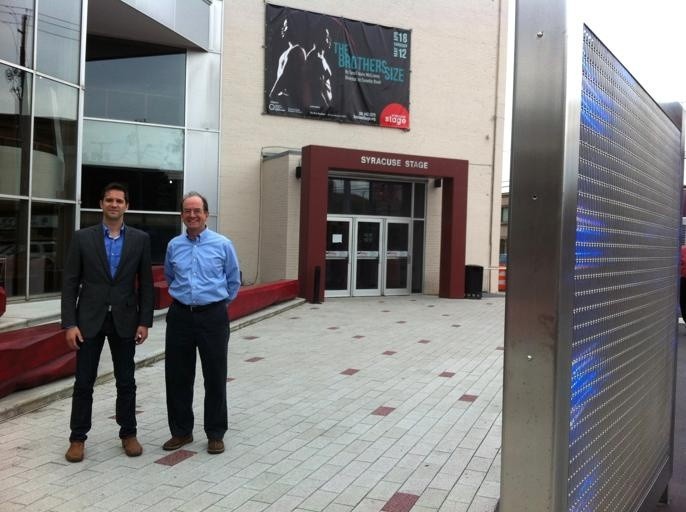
[{"x1": 464, "y1": 266, "x2": 483, "y2": 298}]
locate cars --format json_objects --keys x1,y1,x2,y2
[{"x1": 0, "y1": 241, "x2": 57, "y2": 279}]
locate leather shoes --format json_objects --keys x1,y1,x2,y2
[
  {"x1": 123, "y1": 436, "x2": 143, "y2": 457},
  {"x1": 207, "y1": 438, "x2": 225, "y2": 454},
  {"x1": 66, "y1": 443, "x2": 84, "y2": 463},
  {"x1": 162, "y1": 433, "x2": 193, "y2": 451}
]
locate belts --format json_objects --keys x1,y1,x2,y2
[{"x1": 177, "y1": 301, "x2": 217, "y2": 312}]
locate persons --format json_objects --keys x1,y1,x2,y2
[
  {"x1": 162, "y1": 192, "x2": 241, "y2": 454},
  {"x1": 267, "y1": 14, "x2": 335, "y2": 115},
  {"x1": 60, "y1": 182, "x2": 155, "y2": 463}
]
